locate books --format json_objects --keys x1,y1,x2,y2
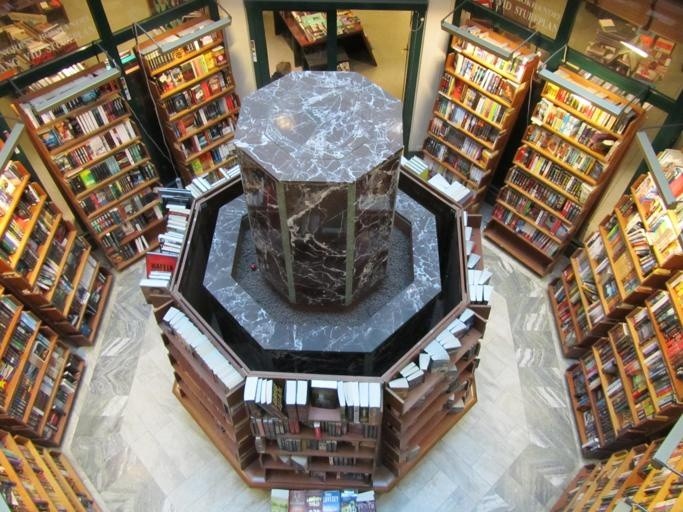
[{"x1": 0, "y1": 0, "x2": 683, "y2": 512}]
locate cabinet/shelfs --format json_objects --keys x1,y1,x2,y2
[
  {"x1": 133, "y1": 0, "x2": 249, "y2": 201},
  {"x1": 422, "y1": 17, "x2": 541, "y2": 222},
  {"x1": 481, "y1": 66, "x2": 649, "y2": 280},
  {"x1": 0, "y1": 139, "x2": 117, "y2": 512},
  {"x1": 10, "y1": 40, "x2": 175, "y2": 272},
  {"x1": 544, "y1": 144, "x2": 683, "y2": 512},
  {"x1": 138, "y1": 149, "x2": 495, "y2": 494}
]
